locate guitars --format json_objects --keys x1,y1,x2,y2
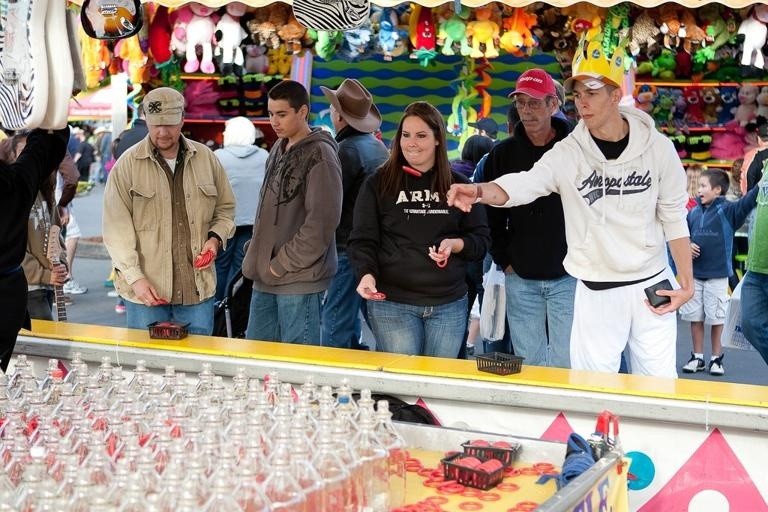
[{"x1": 44, "y1": 225, "x2": 67, "y2": 322}]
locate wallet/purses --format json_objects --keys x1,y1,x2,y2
[{"x1": 644, "y1": 279, "x2": 673, "y2": 308}]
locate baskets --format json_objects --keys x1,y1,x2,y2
[
  {"x1": 440, "y1": 440, "x2": 522, "y2": 491},
  {"x1": 472, "y1": 352, "x2": 526, "y2": 376},
  {"x1": 147, "y1": 321, "x2": 191, "y2": 340}
]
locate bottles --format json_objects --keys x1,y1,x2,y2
[{"x1": 0, "y1": 352, "x2": 409, "y2": 512}]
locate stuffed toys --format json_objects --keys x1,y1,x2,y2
[
  {"x1": 632, "y1": 86, "x2": 767, "y2": 164},
  {"x1": 73, "y1": 0, "x2": 768, "y2": 86}
]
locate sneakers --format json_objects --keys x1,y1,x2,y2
[
  {"x1": 62, "y1": 278, "x2": 88, "y2": 295},
  {"x1": 682, "y1": 351, "x2": 706, "y2": 372},
  {"x1": 52, "y1": 294, "x2": 73, "y2": 305},
  {"x1": 709, "y1": 353, "x2": 724, "y2": 375}
]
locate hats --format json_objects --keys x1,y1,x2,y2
[
  {"x1": 563, "y1": 75, "x2": 607, "y2": 93},
  {"x1": 319, "y1": 78, "x2": 383, "y2": 133},
  {"x1": 467, "y1": 118, "x2": 498, "y2": 135},
  {"x1": 142, "y1": 87, "x2": 186, "y2": 126},
  {"x1": 508, "y1": 68, "x2": 556, "y2": 100}
]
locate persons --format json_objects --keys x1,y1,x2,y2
[
  {"x1": 0, "y1": 130, "x2": 70, "y2": 325},
  {"x1": 1, "y1": 123, "x2": 72, "y2": 373},
  {"x1": 101, "y1": 68, "x2": 575, "y2": 371},
  {"x1": 445, "y1": 41, "x2": 695, "y2": 381},
  {"x1": 49, "y1": 123, "x2": 115, "y2": 305},
  {"x1": 670, "y1": 118, "x2": 768, "y2": 378}
]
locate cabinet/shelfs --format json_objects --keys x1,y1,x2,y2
[
  {"x1": 167, "y1": 74, "x2": 290, "y2": 125},
  {"x1": 635, "y1": 79, "x2": 768, "y2": 168}
]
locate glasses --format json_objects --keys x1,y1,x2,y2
[{"x1": 512, "y1": 98, "x2": 544, "y2": 109}]
